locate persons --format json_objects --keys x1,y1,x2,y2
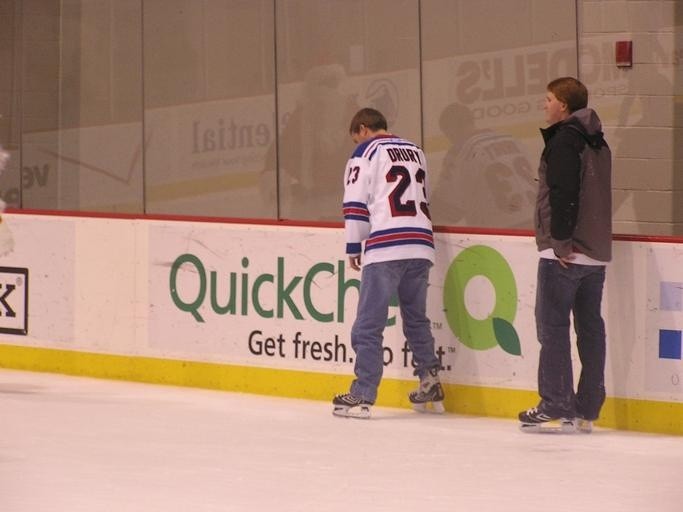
[
  {"x1": 517, "y1": 77, "x2": 612, "y2": 423},
  {"x1": 332, "y1": 108, "x2": 444, "y2": 407},
  {"x1": 429, "y1": 104, "x2": 540, "y2": 231}
]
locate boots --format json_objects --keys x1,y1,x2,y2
[
  {"x1": 409, "y1": 367, "x2": 444, "y2": 403},
  {"x1": 333, "y1": 393, "x2": 372, "y2": 409}
]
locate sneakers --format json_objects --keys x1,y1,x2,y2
[{"x1": 518, "y1": 402, "x2": 601, "y2": 422}]
